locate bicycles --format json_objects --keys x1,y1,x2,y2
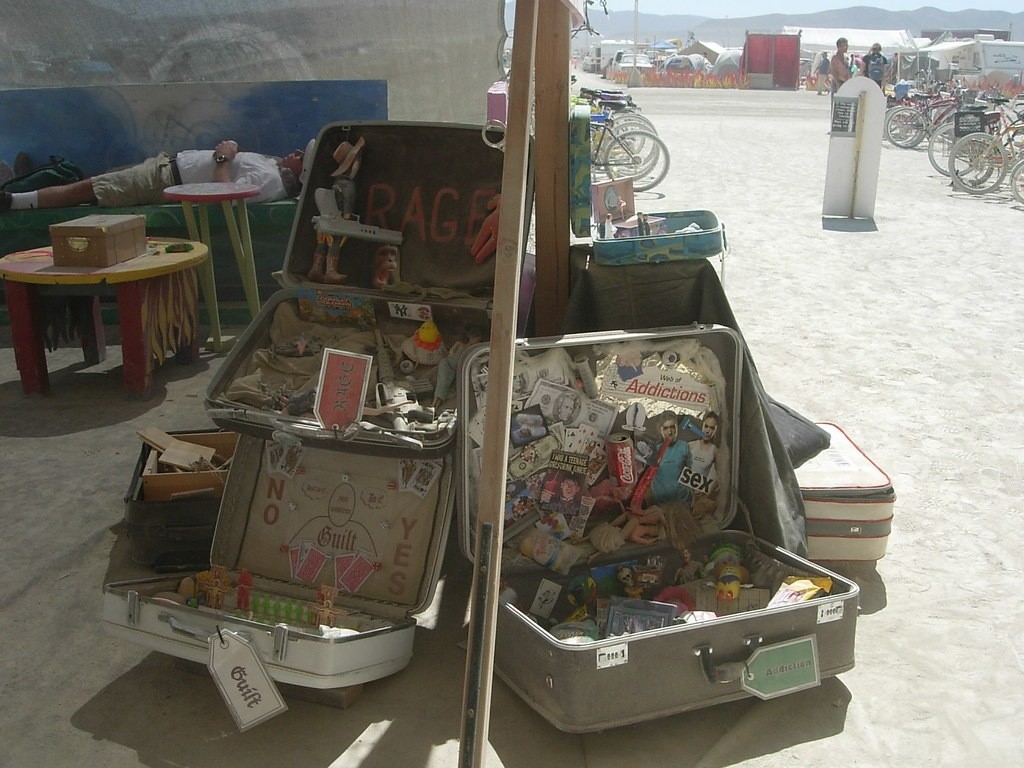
[
  {"x1": 571, "y1": 76, "x2": 671, "y2": 193},
  {"x1": 884, "y1": 69, "x2": 1024, "y2": 205}
]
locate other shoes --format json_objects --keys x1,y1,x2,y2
[{"x1": 0, "y1": 192, "x2": 13, "y2": 211}]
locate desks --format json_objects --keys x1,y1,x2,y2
[{"x1": 0, "y1": 236, "x2": 208, "y2": 400}]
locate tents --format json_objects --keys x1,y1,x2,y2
[
  {"x1": 779, "y1": 25, "x2": 978, "y2": 89},
  {"x1": 648, "y1": 39, "x2": 742, "y2": 76}
]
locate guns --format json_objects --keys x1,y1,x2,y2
[
  {"x1": 375, "y1": 328, "x2": 435, "y2": 450},
  {"x1": 311, "y1": 188, "x2": 406, "y2": 245}
]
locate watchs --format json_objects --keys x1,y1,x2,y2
[{"x1": 215, "y1": 155, "x2": 232, "y2": 163}]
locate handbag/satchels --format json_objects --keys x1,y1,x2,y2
[{"x1": 2, "y1": 158, "x2": 83, "y2": 193}]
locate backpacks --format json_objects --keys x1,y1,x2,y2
[{"x1": 868, "y1": 55, "x2": 884, "y2": 81}]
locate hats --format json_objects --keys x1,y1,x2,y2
[{"x1": 299, "y1": 139, "x2": 317, "y2": 184}]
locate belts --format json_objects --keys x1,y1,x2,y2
[{"x1": 169, "y1": 153, "x2": 181, "y2": 186}]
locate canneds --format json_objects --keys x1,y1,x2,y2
[{"x1": 606, "y1": 433, "x2": 638, "y2": 488}]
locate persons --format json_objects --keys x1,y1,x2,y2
[
  {"x1": 235, "y1": 566, "x2": 253, "y2": 611},
  {"x1": 307, "y1": 136, "x2": 366, "y2": 284},
  {"x1": 0, "y1": 140, "x2": 306, "y2": 210},
  {"x1": 512, "y1": 367, "x2": 532, "y2": 401},
  {"x1": 540, "y1": 472, "x2": 580, "y2": 516},
  {"x1": 553, "y1": 391, "x2": 581, "y2": 426},
  {"x1": 857, "y1": 43, "x2": 888, "y2": 87},
  {"x1": 637, "y1": 211, "x2": 651, "y2": 236},
  {"x1": 573, "y1": 505, "x2": 704, "y2": 565},
  {"x1": 813, "y1": 51, "x2": 831, "y2": 95},
  {"x1": 431, "y1": 323, "x2": 482, "y2": 411},
  {"x1": 604, "y1": 213, "x2": 617, "y2": 239},
  {"x1": 672, "y1": 546, "x2": 704, "y2": 586},
  {"x1": 651, "y1": 411, "x2": 693, "y2": 511},
  {"x1": 688, "y1": 411, "x2": 721, "y2": 498},
  {"x1": 831, "y1": 37, "x2": 887, "y2": 135}
]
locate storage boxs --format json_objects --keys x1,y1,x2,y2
[
  {"x1": 143, "y1": 431, "x2": 237, "y2": 500},
  {"x1": 591, "y1": 176, "x2": 668, "y2": 237},
  {"x1": 49, "y1": 213, "x2": 147, "y2": 267}
]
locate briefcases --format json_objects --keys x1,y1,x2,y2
[{"x1": 97, "y1": 104, "x2": 902, "y2": 735}]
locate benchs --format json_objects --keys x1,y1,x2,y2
[{"x1": 0, "y1": 198, "x2": 298, "y2": 327}]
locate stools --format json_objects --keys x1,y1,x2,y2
[{"x1": 162, "y1": 181, "x2": 261, "y2": 352}]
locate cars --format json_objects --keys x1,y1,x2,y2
[
  {"x1": 582, "y1": 40, "x2": 654, "y2": 78},
  {"x1": 645, "y1": 50, "x2": 662, "y2": 66}
]
